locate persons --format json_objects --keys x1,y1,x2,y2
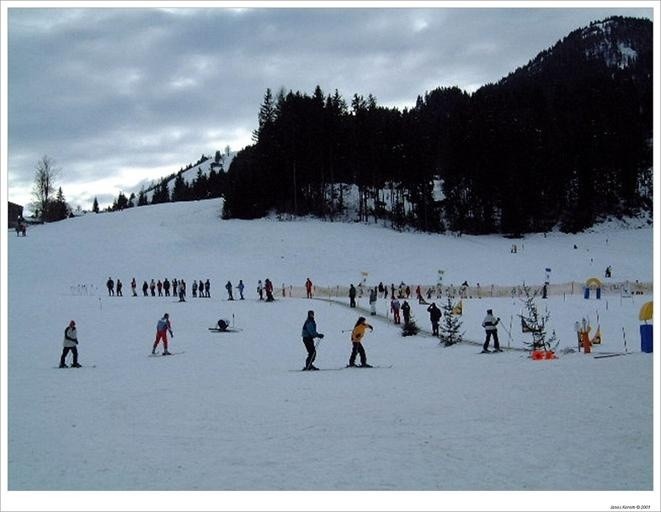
[
  {"x1": 59, "y1": 320, "x2": 81, "y2": 368},
  {"x1": 106, "y1": 277, "x2": 210, "y2": 302},
  {"x1": 349, "y1": 284, "x2": 356, "y2": 308},
  {"x1": 348, "y1": 317, "x2": 374, "y2": 367},
  {"x1": 225, "y1": 281, "x2": 234, "y2": 300},
  {"x1": 606, "y1": 265, "x2": 612, "y2": 277},
  {"x1": 368, "y1": 281, "x2": 481, "y2": 338},
  {"x1": 306, "y1": 278, "x2": 313, "y2": 299},
  {"x1": 510, "y1": 244, "x2": 517, "y2": 253},
  {"x1": 635, "y1": 280, "x2": 644, "y2": 295},
  {"x1": 237, "y1": 280, "x2": 245, "y2": 300},
  {"x1": 256, "y1": 279, "x2": 275, "y2": 302},
  {"x1": 481, "y1": 309, "x2": 504, "y2": 352},
  {"x1": 542, "y1": 285, "x2": 548, "y2": 298},
  {"x1": 152, "y1": 313, "x2": 173, "y2": 355},
  {"x1": 302, "y1": 311, "x2": 324, "y2": 370}
]
